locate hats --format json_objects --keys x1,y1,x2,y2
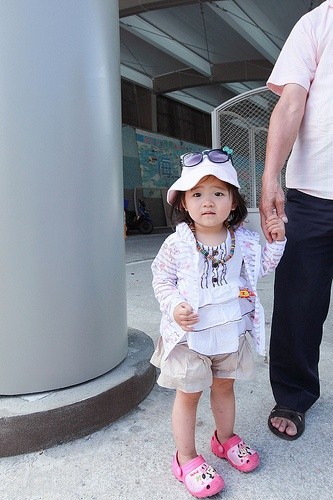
[{"x1": 166, "y1": 154, "x2": 241, "y2": 209}]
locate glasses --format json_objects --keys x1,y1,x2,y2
[{"x1": 180, "y1": 148, "x2": 233, "y2": 167}]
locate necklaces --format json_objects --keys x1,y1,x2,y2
[{"x1": 189, "y1": 221, "x2": 235, "y2": 264}]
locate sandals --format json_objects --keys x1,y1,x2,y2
[
  {"x1": 210, "y1": 429, "x2": 259, "y2": 472},
  {"x1": 268, "y1": 404, "x2": 305, "y2": 440},
  {"x1": 172, "y1": 451, "x2": 225, "y2": 498}
]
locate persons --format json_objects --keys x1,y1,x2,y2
[
  {"x1": 259, "y1": 0, "x2": 333, "y2": 441},
  {"x1": 151, "y1": 148, "x2": 288, "y2": 498}
]
